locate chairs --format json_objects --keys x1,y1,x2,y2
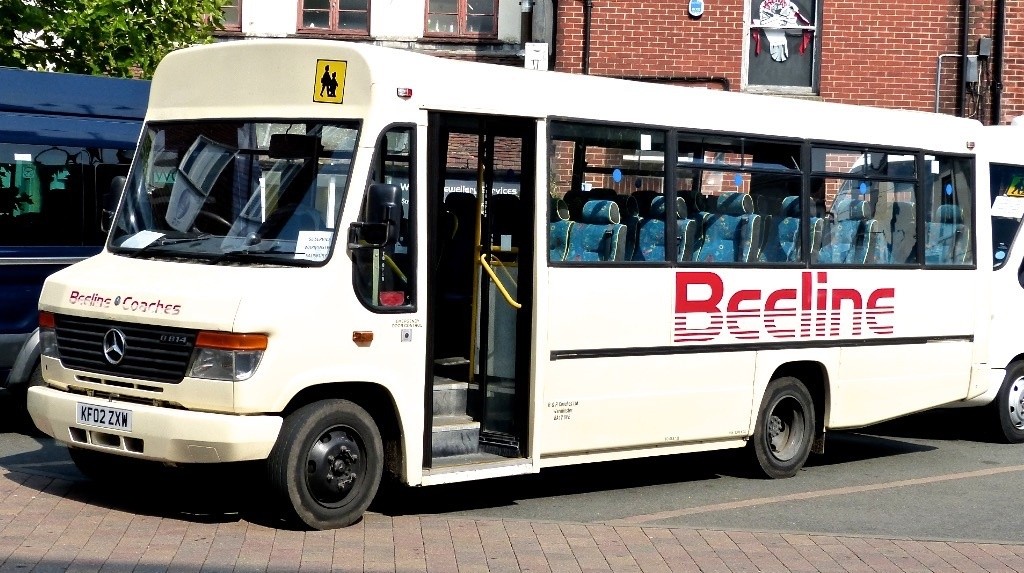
[{"x1": 445, "y1": 191, "x2": 969, "y2": 263}]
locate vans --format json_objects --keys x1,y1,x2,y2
[{"x1": 0, "y1": 67, "x2": 265, "y2": 393}]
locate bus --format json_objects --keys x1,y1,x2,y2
[
  {"x1": 25, "y1": 28, "x2": 992, "y2": 530},
  {"x1": 819, "y1": 115, "x2": 1024, "y2": 446}
]
